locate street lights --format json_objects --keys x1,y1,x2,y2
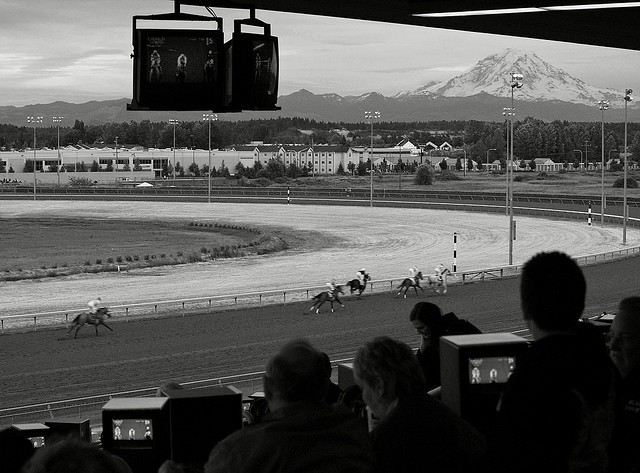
[
  {"x1": 169, "y1": 118, "x2": 178, "y2": 178},
  {"x1": 364, "y1": 109, "x2": 381, "y2": 206},
  {"x1": 598, "y1": 99, "x2": 611, "y2": 227},
  {"x1": 28, "y1": 116, "x2": 43, "y2": 199},
  {"x1": 203, "y1": 113, "x2": 218, "y2": 203},
  {"x1": 53, "y1": 115, "x2": 63, "y2": 185},
  {"x1": 502, "y1": 107, "x2": 516, "y2": 216}
]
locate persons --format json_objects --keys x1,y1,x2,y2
[
  {"x1": 143, "y1": 426, "x2": 152, "y2": 439},
  {"x1": 324, "y1": 280, "x2": 336, "y2": 298},
  {"x1": 321, "y1": 351, "x2": 342, "y2": 404},
  {"x1": 607, "y1": 297, "x2": 640, "y2": 473},
  {"x1": 409, "y1": 302, "x2": 483, "y2": 397},
  {"x1": 432, "y1": 263, "x2": 446, "y2": 280},
  {"x1": 489, "y1": 369, "x2": 497, "y2": 382},
  {"x1": 204, "y1": 337, "x2": 367, "y2": 473},
  {"x1": 488, "y1": 252, "x2": 625, "y2": 473},
  {"x1": 85, "y1": 298, "x2": 103, "y2": 314},
  {"x1": 352, "y1": 336, "x2": 490, "y2": 473},
  {"x1": 407, "y1": 265, "x2": 418, "y2": 283},
  {"x1": 508, "y1": 357, "x2": 516, "y2": 378},
  {"x1": 471, "y1": 367, "x2": 481, "y2": 382},
  {"x1": 128, "y1": 427, "x2": 135, "y2": 440},
  {"x1": 30, "y1": 437, "x2": 45, "y2": 448},
  {"x1": 356, "y1": 267, "x2": 366, "y2": 284},
  {"x1": 114, "y1": 427, "x2": 122, "y2": 439}
]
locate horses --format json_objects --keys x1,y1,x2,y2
[
  {"x1": 65, "y1": 306, "x2": 115, "y2": 338},
  {"x1": 397, "y1": 271, "x2": 424, "y2": 297},
  {"x1": 423, "y1": 268, "x2": 452, "y2": 294},
  {"x1": 345, "y1": 272, "x2": 371, "y2": 294},
  {"x1": 309, "y1": 284, "x2": 345, "y2": 314}
]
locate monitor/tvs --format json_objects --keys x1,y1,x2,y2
[
  {"x1": 212, "y1": 32, "x2": 279, "y2": 111},
  {"x1": 133, "y1": 28, "x2": 224, "y2": 107}
]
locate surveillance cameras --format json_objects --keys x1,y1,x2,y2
[{"x1": 517, "y1": 73, "x2": 523, "y2": 79}]
